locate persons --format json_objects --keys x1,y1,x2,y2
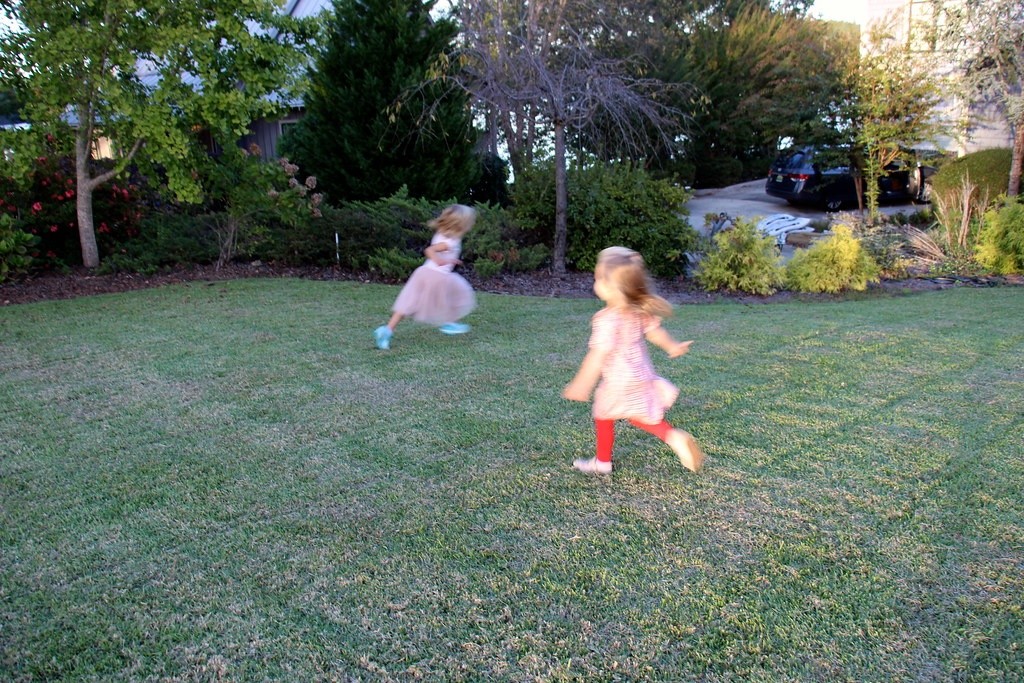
[
  {"x1": 561, "y1": 246, "x2": 701, "y2": 473},
  {"x1": 372, "y1": 204, "x2": 478, "y2": 350}
]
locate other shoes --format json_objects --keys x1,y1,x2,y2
[
  {"x1": 373, "y1": 326, "x2": 393, "y2": 351},
  {"x1": 573, "y1": 455, "x2": 612, "y2": 475},
  {"x1": 668, "y1": 428, "x2": 701, "y2": 474},
  {"x1": 439, "y1": 322, "x2": 471, "y2": 334}
]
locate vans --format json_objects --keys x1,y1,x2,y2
[{"x1": 765, "y1": 143, "x2": 939, "y2": 211}]
